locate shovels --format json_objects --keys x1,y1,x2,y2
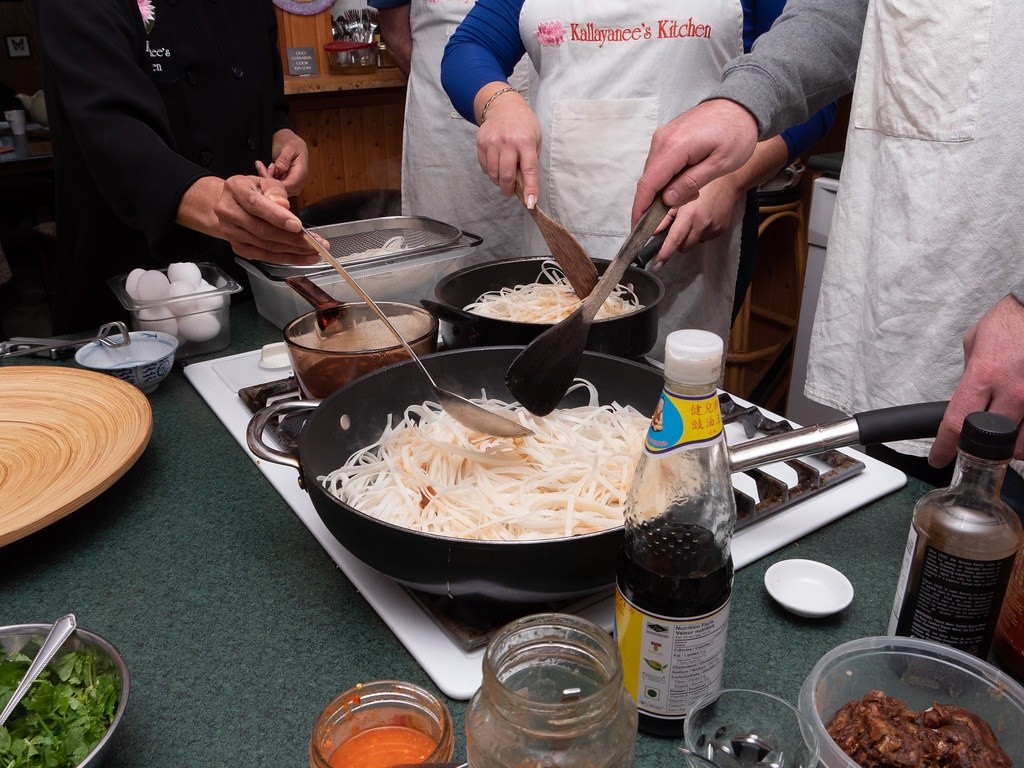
[
  {"x1": 505, "y1": 190, "x2": 672, "y2": 417},
  {"x1": 513, "y1": 181, "x2": 599, "y2": 301}
]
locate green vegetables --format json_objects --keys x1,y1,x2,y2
[{"x1": 0, "y1": 645, "x2": 116, "y2": 768}]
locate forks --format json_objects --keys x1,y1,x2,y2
[{"x1": 344, "y1": 9, "x2": 380, "y2": 43}]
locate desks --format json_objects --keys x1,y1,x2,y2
[{"x1": 0, "y1": 128, "x2": 55, "y2": 172}]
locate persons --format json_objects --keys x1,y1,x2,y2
[
  {"x1": 439, "y1": 0, "x2": 845, "y2": 398},
  {"x1": 39, "y1": 0, "x2": 332, "y2": 341},
  {"x1": 631, "y1": 1, "x2": 1024, "y2": 488},
  {"x1": 368, "y1": 0, "x2": 545, "y2": 267}
]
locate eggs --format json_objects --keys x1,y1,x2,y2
[{"x1": 125, "y1": 263, "x2": 224, "y2": 342}]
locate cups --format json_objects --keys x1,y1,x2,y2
[{"x1": 4, "y1": 110, "x2": 26, "y2": 135}]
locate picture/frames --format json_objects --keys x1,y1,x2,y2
[{"x1": 3, "y1": 33, "x2": 33, "y2": 58}]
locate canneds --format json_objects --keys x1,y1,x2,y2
[{"x1": 309, "y1": 680, "x2": 454, "y2": 768}]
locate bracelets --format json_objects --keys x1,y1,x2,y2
[{"x1": 481, "y1": 87, "x2": 529, "y2": 122}]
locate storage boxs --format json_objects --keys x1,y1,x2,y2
[{"x1": 235, "y1": 237, "x2": 477, "y2": 332}]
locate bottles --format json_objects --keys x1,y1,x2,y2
[
  {"x1": 992, "y1": 527, "x2": 1024, "y2": 683},
  {"x1": 307, "y1": 677, "x2": 454, "y2": 766},
  {"x1": 885, "y1": 408, "x2": 1020, "y2": 684},
  {"x1": 613, "y1": 322, "x2": 735, "y2": 740},
  {"x1": 464, "y1": 605, "x2": 637, "y2": 765}
]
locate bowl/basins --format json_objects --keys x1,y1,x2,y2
[
  {"x1": 327, "y1": 45, "x2": 379, "y2": 74},
  {"x1": 799, "y1": 636, "x2": 1024, "y2": 768},
  {"x1": 73, "y1": 331, "x2": 181, "y2": 395},
  {"x1": 0, "y1": 623, "x2": 131, "y2": 768},
  {"x1": 683, "y1": 688, "x2": 820, "y2": 768}
]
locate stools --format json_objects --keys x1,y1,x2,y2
[{"x1": 722, "y1": 193, "x2": 808, "y2": 401}]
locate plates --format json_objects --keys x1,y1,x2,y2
[
  {"x1": 760, "y1": 555, "x2": 853, "y2": 622},
  {"x1": 0, "y1": 366, "x2": 152, "y2": 558}
]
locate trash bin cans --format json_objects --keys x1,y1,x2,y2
[{"x1": 297, "y1": 189, "x2": 401, "y2": 229}]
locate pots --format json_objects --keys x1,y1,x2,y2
[
  {"x1": 421, "y1": 217, "x2": 673, "y2": 347},
  {"x1": 245, "y1": 341, "x2": 951, "y2": 578},
  {"x1": 281, "y1": 275, "x2": 441, "y2": 394}
]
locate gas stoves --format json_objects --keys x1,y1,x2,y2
[{"x1": 184, "y1": 315, "x2": 907, "y2": 701}]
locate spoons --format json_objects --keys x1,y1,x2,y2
[{"x1": 330, "y1": 15, "x2": 351, "y2": 42}]
